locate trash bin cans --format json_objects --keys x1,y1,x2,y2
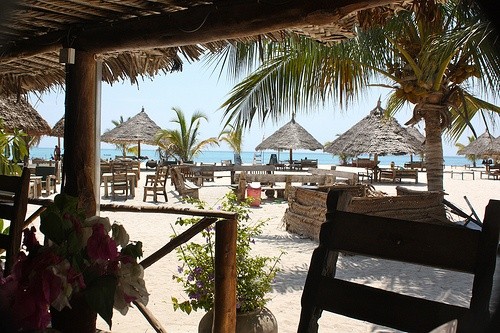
[{"x1": 245, "y1": 182, "x2": 262, "y2": 206}]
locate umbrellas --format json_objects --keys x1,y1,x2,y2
[
  {"x1": 0, "y1": 89, "x2": 65, "y2": 162},
  {"x1": 255, "y1": 113, "x2": 324, "y2": 164},
  {"x1": 325, "y1": 100, "x2": 500, "y2": 182},
  {"x1": 101, "y1": 107, "x2": 167, "y2": 160}
]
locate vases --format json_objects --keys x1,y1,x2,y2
[{"x1": 198, "y1": 306, "x2": 278, "y2": 333}]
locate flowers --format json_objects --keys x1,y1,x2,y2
[
  {"x1": 169, "y1": 191, "x2": 289, "y2": 316},
  {"x1": 0, "y1": 194, "x2": 150, "y2": 333}
]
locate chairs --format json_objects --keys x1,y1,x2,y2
[
  {"x1": 111, "y1": 166, "x2": 128, "y2": 201},
  {"x1": 200, "y1": 162, "x2": 217, "y2": 182},
  {"x1": 0, "y1": 167, "x2": 30, "y2": 278},
  {"x1": 181, "y1": 161, "x2": 197, "y2": 180},
  {"x1": 131, "y1": 160, "x2": 140, "y2": 180},
  {"x1": 297, "y1": 189, "x2": 500, "y2": 333},
  {"x1": 358, "y1": 167, "x2": 373, "y2": 184},
  {"x1": 143, "y1": 164, "x2": 170, "y2": 203},
  {"x1": 486, "y1": 165, "x2": 498, "y2": 180}
]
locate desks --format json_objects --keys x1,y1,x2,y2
[
  {"x1": 30, "y1": 176, "x2": 43, "y2": 199},
  {"x1": 102, "y1": 173, "x2": 136, "y2": 198},
  {"x1": 170, "y1": 159, "x2": 299, "y2": 182}
]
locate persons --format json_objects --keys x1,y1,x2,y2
[{"x1": 54, "y1": 145, "x2": 60, "y2": 162}]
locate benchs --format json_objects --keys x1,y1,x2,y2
[
  {"x1": 230, "y1": 165, "x2": 276, "y2": 188},
  {"x1": 379, "y1": 169, "x2": 418, "y2": 184},
  {"x1": 301, "y1": 159, "x2": 318, "y2": 171},
  {"x1": 171, "y1": 167, "x2": 200, "y2": 200},
  {"x1": 28, "y1": 166, "x2": 59, "y2": 197}
]
